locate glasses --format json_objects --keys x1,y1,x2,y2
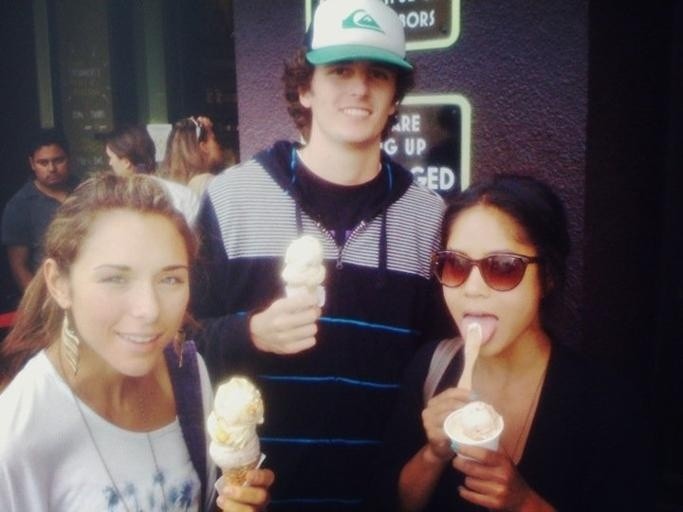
[{"x1": 430, "y1": 252, "x2": 547, "y2": 292}]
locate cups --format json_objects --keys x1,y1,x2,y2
[{"x1": 443, "y1": 408, "x2": 506, "y2": 465}]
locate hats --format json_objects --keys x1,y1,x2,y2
[{"x1": 305, "y1": 0, "x2": 414, "y2": 70}]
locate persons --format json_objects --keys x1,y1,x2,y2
[
  {"x1": 361, "y1": 174, "x2": 659, "y2": 512},
  {"x1": 181, "y1": 0, "x2": 448, "y2": 511},
  {"x1": 4, "y1": 129, "x2": 79, "y2": 297},
  {"x1": 103, "y1": 125, "x2": 157, "y2": 178},
  {"x1": 163, "y1": 115, "x2": 225, "y2": 198},
  {"x1": 1, "y1": 167, "x2": 279, "y2": 510}
]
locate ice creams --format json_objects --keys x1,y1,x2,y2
[
  {"x1": 206, "y1": 375, "x2": 265, "y2": 486},
  {"x1": 282, "y1": 234, "x2": 327, "y2": 301},
  {"x1": 445, "y1": 399, "x2": 504, "y2": 459}
]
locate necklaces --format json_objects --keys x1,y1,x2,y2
[{"x1": 63, "y1": 373, "x2": 167, "y2": 511}]
